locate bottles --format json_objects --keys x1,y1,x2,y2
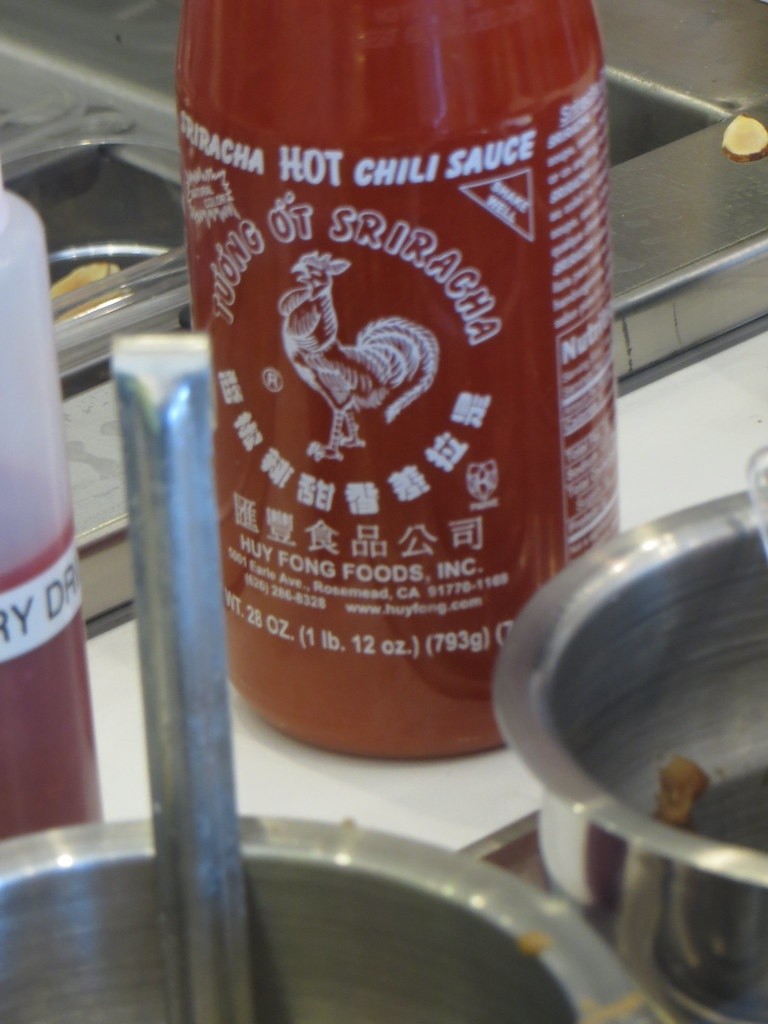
[
  {"x1": 179, "y1": 0, "x2": 616, "y2": 765},
  {"x1": 2, "y1": 181, "x2": 105, "y2": 831}
]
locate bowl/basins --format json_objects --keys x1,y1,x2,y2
[
  {"x1": 46, "y1": 243, "x2": 193, "y2": 402},
  {"x1": 0, "y1": 814, "x2": 666, "y2": 1024},
  {"x1": 493, "y1": 488, "x2": 768, "y2": 1024}
]
